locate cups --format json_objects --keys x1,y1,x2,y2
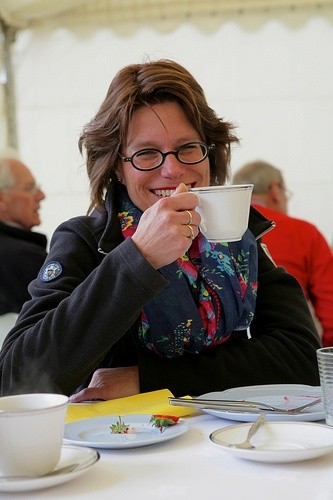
[
  {"x1": 0, "y1": 393, "x2": 70, "y2": 481},
  {"x1": 189, "y1": 184, "x2": 254, "y2": 242},
  {"x1": 316, "y1": 347, "x2": 333, "y2": 427}
]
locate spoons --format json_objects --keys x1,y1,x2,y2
[
  {"x1": 0, "y1": 463, "x2": 78, "y2": 480},
  {"x1": 228, "y1": 411, "x2": 266, "y2": 450}
]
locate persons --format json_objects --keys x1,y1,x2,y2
[
  {"x1": 0, "y1": 157, "x2": 57, "y2": 323},
  {"x1": 0, "y1": 57, "x2": 328, "y2": 404},
  {"x1": 226, "y1": 161, "x2": 333, "y2": 358}
]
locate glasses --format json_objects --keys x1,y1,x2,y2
[
  {"x1": 117, "y1": 141, "x2": 215, "y2": 171},
  {"x1": 267, "y1": 181, "x2": 292, "y2": 202}
]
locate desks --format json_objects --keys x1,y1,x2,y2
[{"x1": 1, "y1": 400, "x2": 332, "y2": 500}]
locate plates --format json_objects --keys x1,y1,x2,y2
[
  {"x1": 208, "y1": 421, "x2": 333, "y2": 463},
  {"x1": 61, "y1": 413, "x2": 190, "y2": 449},
  {"x1": 201, "y1": 394, "x2": 324, "y2": 422},
  {"x1": 0, "y1": 446, "x2": 101, "y2": 493}
]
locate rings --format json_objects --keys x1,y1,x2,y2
[
  {"x1": 183, "y1": 209, "x2": 193, "y2": 225},
  {"x1": 185, "y1": 224, "x2": 195, "y2": 240}
]
